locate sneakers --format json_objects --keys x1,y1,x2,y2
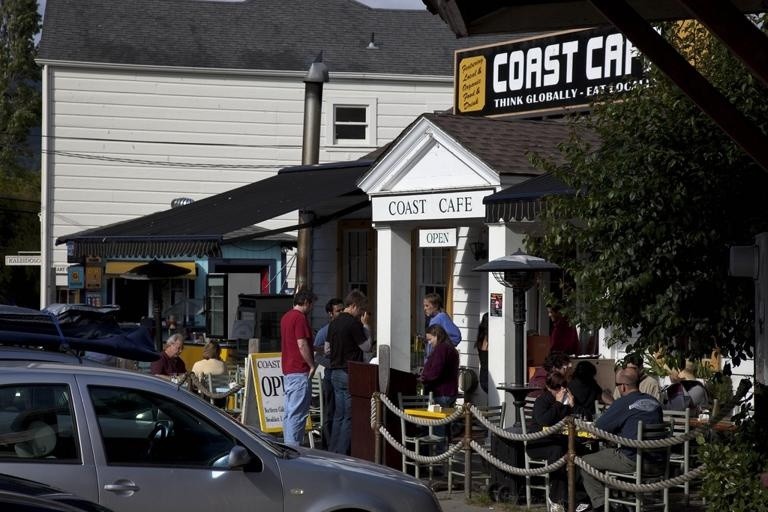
[
  {"x1": 548, "y1": 498, "x2": 565, "y2": 512},
  {"x1": 576, "y1": 503, "x2": 590, "y2": 512}
]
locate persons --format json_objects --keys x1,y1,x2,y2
[
  {"x1": 476, "y1": 312, "x2": 488, "y2": 394},
  {"x1": 281, "y1": 290, "x2": 315, "y2": 447},
  {"x1": 423, "y1": 293, "x2": 462, "y2": 367},
  {"x1": 191, "y1": 340, "x2": 227, "y2": 408},
  {"x1": 314, "y1": 298, "x2": 346, "y2": 452},
  {"x1": 420, "y1": 324, "x2": 459, "y2": 493},
  {"x1": 546, "y1": 304, "x2": 578, "y2": 354},
  {"x1": 151, "y1": 333, "x2": 187, "y2": 375},
  {"x1": 324, "y1": 289, "x2": 373, "y2": 455},
  {"x1": 530, "y1": 350, "x2": 708, "y2": 512}
]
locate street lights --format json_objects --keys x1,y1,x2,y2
[
  {"x1": 473, "y1": 246, "x2": 563, "y2": 506},
  {"x1": 119, "y1": 257, "x2": 192, "y2": 356}
]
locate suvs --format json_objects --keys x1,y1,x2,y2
[{"x1": 0, "y1": 303, "x2": 444, "y2": 511}]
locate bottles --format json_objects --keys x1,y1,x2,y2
[
  {"x1": 192, "y1": 331, "x2": 196, "y2": 344},
  {"x1": 427, "y1": 390, "x2": 435, "y2": 412},
  {"x1": 198, "y1": 331, "x2": 205, "y2": 344}
]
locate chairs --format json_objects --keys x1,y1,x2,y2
[
  {"x1": 395, "y1": 364, "x2": 717, "y2": 511},
  {"x1": 200, "y1": 363, "x2": 326, "y2": 449},
  {"x1": 15, "y1": 419, "x2": 58, "y2": 458}
]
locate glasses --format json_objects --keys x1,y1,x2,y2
[{"x1": 552, "y1": 382, "x2": 568, "y2": 390}]
